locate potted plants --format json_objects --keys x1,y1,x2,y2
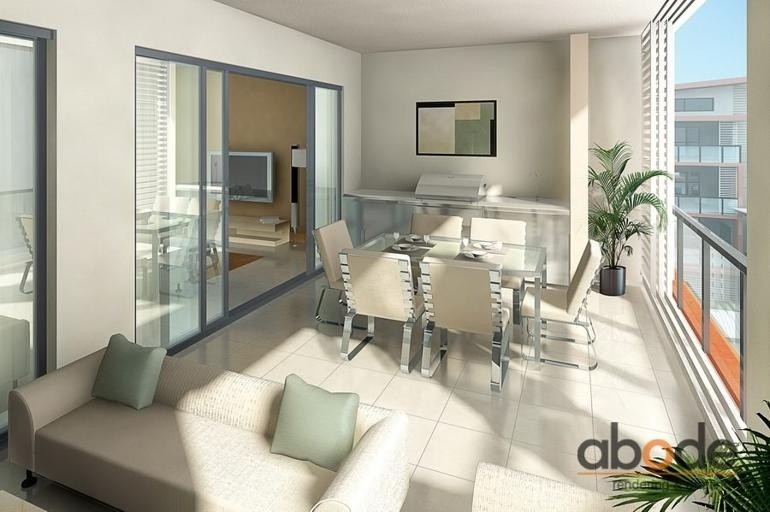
[{"x1": 587, "y1": 135, "x2": 676, "y2": 301}]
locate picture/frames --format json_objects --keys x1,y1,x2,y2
[{"x1": 415, "y1": 98, "x2": 498, "y2": 160}]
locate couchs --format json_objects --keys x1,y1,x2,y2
[{"x1": 5, "y1": 337, "x2": 418, "y2": 512}]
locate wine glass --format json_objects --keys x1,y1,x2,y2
[
  {"x1": 393, "y1": 232, "x2": 399, "y2": 244},
  {"x1": 424, "y1": 235, "x2": 429, "y2": 246}
]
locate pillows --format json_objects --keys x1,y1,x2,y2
[
  {"x1": 89, "y1": 330, "x2": 171, "y2": 413},
  {"x1": 267, "y1": 373, "x2": 360, "y2": 473}
]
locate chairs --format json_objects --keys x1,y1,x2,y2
[{"x1": 12, "y1": 189, "x2": 225, "y2": 311}]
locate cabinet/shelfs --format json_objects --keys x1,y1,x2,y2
[{"x1": 227, "y1": 213, "x2": 292, "y2": 259}]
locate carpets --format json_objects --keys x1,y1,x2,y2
[{"x1": 470, "y1": 460, "x2": 671, "y2": 512}]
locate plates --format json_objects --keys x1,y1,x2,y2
[
  {"x1": 405, "y1": 236, "x2": 424, "y2": 244},
  {"x1": 391, "y1": 243, "x2": 416, "y2": 253},
  {"x1": 459, "y1": 237, "x2": 502, "y2": 261}
]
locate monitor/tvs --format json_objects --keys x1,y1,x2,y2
[{"x1": 207, "y1": 151, "x2": 274, "y2": 203}]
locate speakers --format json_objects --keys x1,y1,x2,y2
[{"x1": 290, "y1": 144, "x2": 300, "y2": 233}]
